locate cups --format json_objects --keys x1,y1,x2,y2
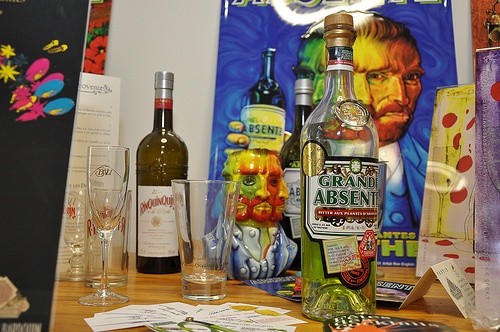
[
  {"x1": 67, "y1": 190, "x2": 131, "y2": 290},
  {"x1": 171, "y1": 180, "x2": 241, "y2": 302}
]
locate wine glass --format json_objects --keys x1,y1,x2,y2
[{"x1": 78, "y1": 146, "x2": 130, "y2": 305}]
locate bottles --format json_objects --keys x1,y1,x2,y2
[
  {"x1": 135, "y1": 71, "x2": 188, "y2": 274},
  {"x1": 279, "y1": 13, "x2": 379, "y2": 322}
]
